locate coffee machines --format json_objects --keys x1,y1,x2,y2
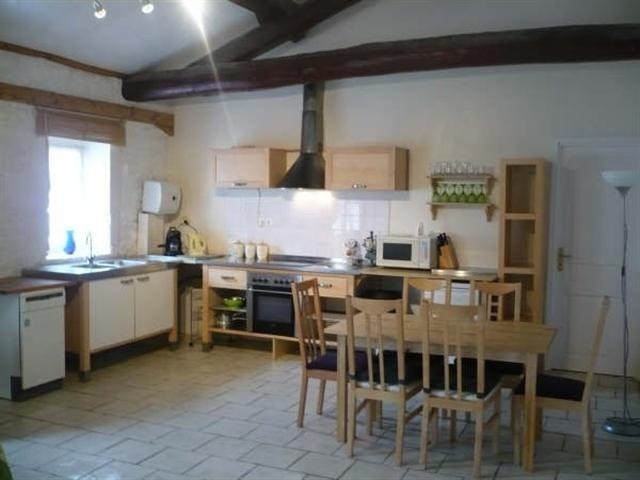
[{"x1": 164, "y1": 226, "x2": 183, "y2": 255}]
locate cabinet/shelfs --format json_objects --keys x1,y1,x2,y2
[
  {"x1": 199, "y1": 263, "x2": 353, "y2": 359},
  {"x1": 324, "y1": 147, "x2": 409, "y2": 192},
  {"x1": 496, "y1": 157, "x2": 552, "y2": 324},
  {"x1": 425, "y1": 174, "x2": 494, "y2": 221},
  {"x1": 212, "y1": 148, "x2": 286, "y2": 189},
  {"x1": 21, "y1": 267, "x2": 180, "y2": 384}
]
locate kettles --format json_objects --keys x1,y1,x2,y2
[{"x1": 190, "y1": 234, "x2": 206, "y2": 257}]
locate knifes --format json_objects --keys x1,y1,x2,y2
[{"x1": 438, "y1": 231, "x2": 447, "y2": 245}]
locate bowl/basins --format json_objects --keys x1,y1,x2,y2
[{"x1": 224, "y1": 296, "x2": 246, "y2": 309}]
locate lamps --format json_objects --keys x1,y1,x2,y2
[
  {"x1": 602, "y1": 171, "x2": 640, "y2": 438},
  {"x1": 138, "y1": 1, "x2": 156, "y2": 16},
  {"x1": 89, "y1": 0, "x2": 107, "y2": 19}
]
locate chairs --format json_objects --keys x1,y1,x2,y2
[
  {"x1": 453, "y1": 278, "x2": 523, "y2": 423},
  {"x1": 345, "y1": 294, "x2": 439, "y2": 468},
  {"x1": 510, "y1": 295, "x2": 610, "y2": 474},
  {"x1": 374, "y1": 273, "x2": 455, "y2": 433},
  {"x1": 290, "y1": 276, "x2": 373, "y2": 439},
  {"x1": 419, "y1": 301, "x2": 505, "y2": 479}
]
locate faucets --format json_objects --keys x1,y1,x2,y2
[{"x1": 84, "y1": 235, "x2": 95, "y2": 264}]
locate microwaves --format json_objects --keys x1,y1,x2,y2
[{"x1": 375, "y1": 234, "x2": 438, "y2": 269}]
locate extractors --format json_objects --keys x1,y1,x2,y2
[{"x1": 275, "y1": 80, "x2": 326, "y2": 191}]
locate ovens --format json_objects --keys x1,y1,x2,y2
[{"x1": 247, "y1": 284, "x2": 301, "y2": 338}]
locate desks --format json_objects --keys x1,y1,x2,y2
[{"x1": 322, "y1": 309, "x2": 564, "y2": 473}]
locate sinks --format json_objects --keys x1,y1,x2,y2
[
  {"x1": 99, "y1": 258, "x2": 145, "y2": 268},
  {"x1": 72, "y1": 263, "x2": 116, "y2": 271}
]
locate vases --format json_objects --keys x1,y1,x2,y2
[{"x1": 63, "y1": 231, "x2": 75, "y2": 254}]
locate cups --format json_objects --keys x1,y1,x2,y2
[{"x1": 430, "y1": 160, "x2": 491, "y2": 177}]
